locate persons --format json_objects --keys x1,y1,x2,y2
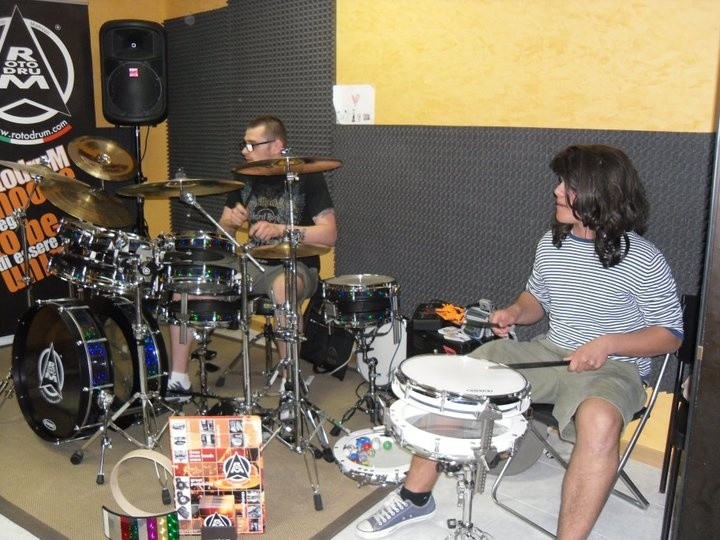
[
  {"x1": 142, "y1": 117, "x2": 338, "y2": 439},
  {"x1": 356, "y1": 145, "x2": 686, "y2": 540}
]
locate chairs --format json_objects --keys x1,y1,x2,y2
[{"x1": 490, "y1": 305, "x2": 686, "y2": 540}]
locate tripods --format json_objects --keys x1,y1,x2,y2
[
  {"x1": 70, "y1": 257, "x2": 184, "y2": 505},
  {"x1": 262, "y1": 259, "x2": 325, "y2": 459},
  {"x1": 262, "y1": 176, "x2": 349, "y2": 462},
  {"x1": 181, "y1": 194, "x2": 271, "y2": 421},
  {"x1": 330, "y1": 328, "x2": 389, "y2": 435}
]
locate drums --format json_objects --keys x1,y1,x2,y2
[
  {"x1": 386, "y1": 353, "x2": 535, "y2": 470},
  {"x1": 320, "y1": 272, "x2": 402, "y2": 330},
  {"x1": 9, "y1": 298, "x2": 175, "y2": 444},
  {"x1": 43, "y1": 212, "x2": 258, "y2": 331}
]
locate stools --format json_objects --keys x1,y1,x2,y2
[{"x1": 211, "y1": 293, "x2": 284, "y2": 390}]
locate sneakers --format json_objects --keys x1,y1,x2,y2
[
  {"x1": 355, "y1": 491, "x2": 437, "y2": 540},
  {"x1": 153, "y1": 380, "x2": 192, "y2": 402}
]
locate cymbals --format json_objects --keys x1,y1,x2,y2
[
  {"x1": 231, "y1": 155, "x2": 345, "y2": 177},
  {"x1": 67, "y1": 133, "x2": 140, "y2": 182},
  {"x1": 114, "y1": 177, "x2": 246, "y2": 199},
  {"x1": 246, "y1": 238, "x2": 333, "y2": 260},
  {"x1": 38, "y1": 176, "x2": 138, "y2": 229},
  {"x1": 0, "y1": 155, "x2": 92, "y2": 187}
]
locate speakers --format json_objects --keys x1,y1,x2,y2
[
  {"x1": 407, "y1": 300, "x2": 450, "y2": 356},
  {"x1": 299, "y1": 278, "x2": 362, "y2": 380},
  {"x1": 99, "y1": 19, "x2": 169, "y2": 127}
]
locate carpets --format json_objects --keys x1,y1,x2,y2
[{"x1": 1, "y1": 318, "x2": 404, "y2": 540}]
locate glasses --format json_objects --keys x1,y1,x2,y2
[{"x1": 238, "y1": 139, "x2": 276, "y2": 152}]
